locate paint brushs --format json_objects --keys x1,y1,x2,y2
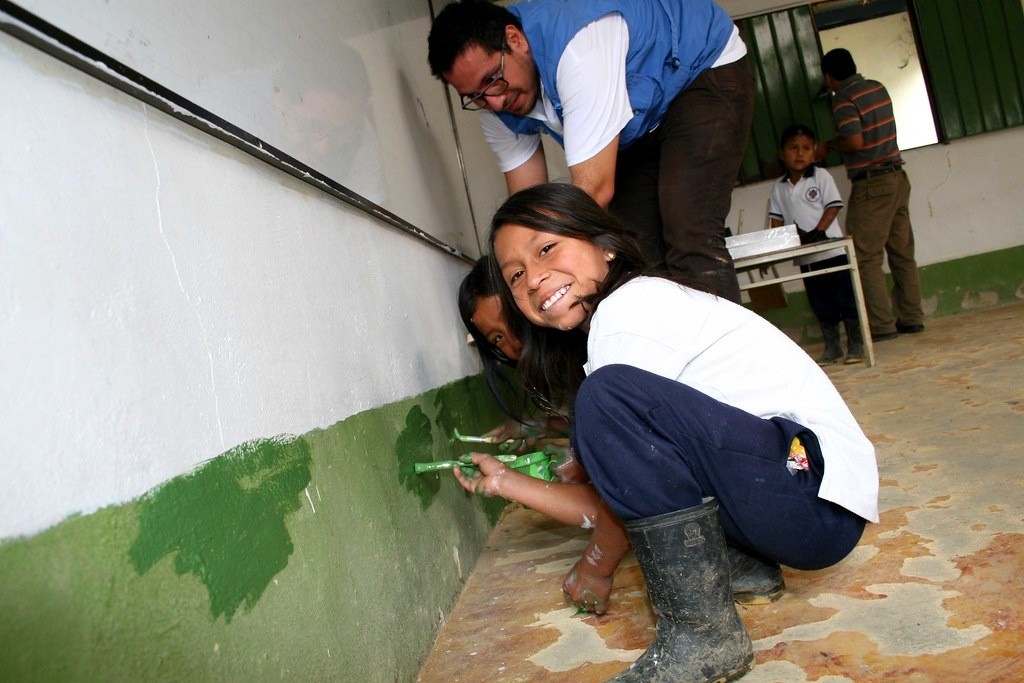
[
  {"x1": 415, "y1": 455, "x2": 517, "y2": 473},
  {"x1": 454, "y1": 428, "x2": 526, "y2": 443}
]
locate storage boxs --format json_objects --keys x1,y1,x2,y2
[{"x1": 725, "y1": 223, "x2": 803, "y2": 261}]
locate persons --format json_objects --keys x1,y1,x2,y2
[
  {"x1": 767, "y1": 129, "x2": 865, "y2": 365},
  {"x1": 453, "y1": 182, "x2": 879, "y2": 683},
  {"x1": 459, "y1": 255, "x2": 605, "y2": 487},
  {"x1": 820, "y1": 48, "x2": 925, "y2": 343},
  {"x1": 427, "y1": 0, "x2": 749, "y2": 308}
]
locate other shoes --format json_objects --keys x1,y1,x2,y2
[
  {"x1": 871, "y1": 331, "x2": 898, "y2": 343},
  {"x1": 895, "y1": 318, "x2": 925, "y2": 334}
]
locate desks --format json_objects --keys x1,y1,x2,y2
[{"x1": 728, "y1": 235, "x2": 877, "y2": 373}]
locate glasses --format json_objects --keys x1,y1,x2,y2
[{"x1": 459, "y1": 39, "x2": 510, "y2": 111}]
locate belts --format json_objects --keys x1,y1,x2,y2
[{"x1": 850, "y1": 164, "x2": 902, "y2": 183}]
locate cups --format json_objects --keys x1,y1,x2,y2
[{"x1": 504, "y1": 452, "x2": 558, "y2": 510}]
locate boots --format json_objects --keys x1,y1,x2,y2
[
  {"x1": 842, "y1": 316, "x2": 867, "y2": 365},
  {"x1": 594, "y1": 493, "x2": 756, "y2": 683},
  {"x1": 725, "y1": 544, "x2": 787, "y2": 606},
  {"x1": 814, "y1": 321, "x2": 845, "y2": 366}
]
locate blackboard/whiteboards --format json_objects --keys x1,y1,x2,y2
[{"x1": 1, "y1": 0, "x2": 483, "y2": 267}]
